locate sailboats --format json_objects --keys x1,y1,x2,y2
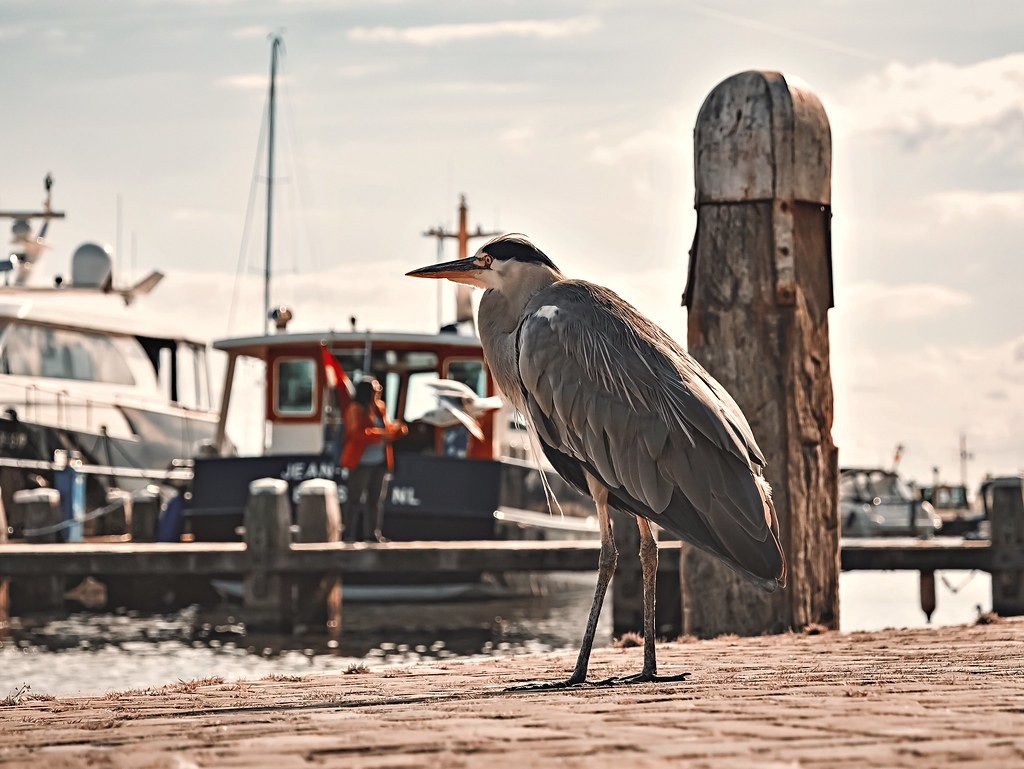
[{"x1": 181, "y1": 34, "x2": 613, "y2": 627}]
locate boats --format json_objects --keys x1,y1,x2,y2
[
  {"x1": 916, "y1": 482, "x2": 992, "y2": 537},
  {"x1": 0, "y1": 170, "x2": 242, "y2": 538},
  {"x1": 833, "y1": 465, "x2": 943, "y2": 537}
]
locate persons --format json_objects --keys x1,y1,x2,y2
[{"x1": 340, "y1": 378, "x2": 408, "y2": 543}]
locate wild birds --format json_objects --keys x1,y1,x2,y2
[{"x1": 405, "y1": 233, "x2": 787, "y2": 691}]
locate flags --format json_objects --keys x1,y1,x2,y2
[{"x1": 318, "y1": 348, "x2": 357, "y2": 402}]
